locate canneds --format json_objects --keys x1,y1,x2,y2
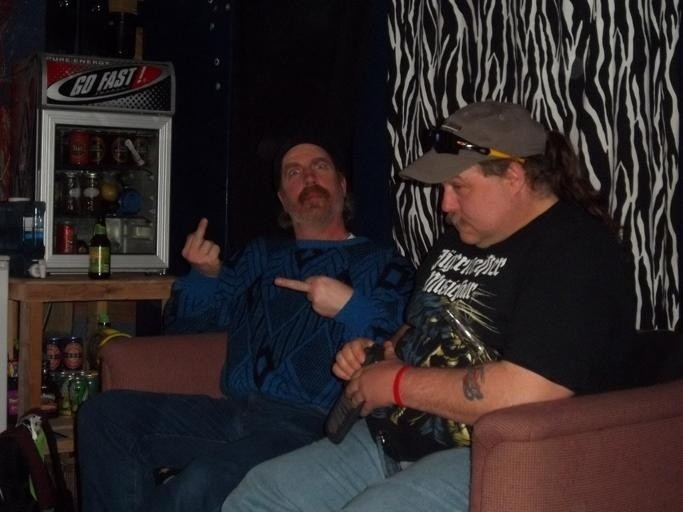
[
  {"x1": 111, "y1": 131, "x2": 129, "y2": 170},
  {"x1": 130, "y1": 134, "x2": 149, "y2": 169},
  {"x1": 43, "y1": 371, "x2": 100, "y2": 417},
  {"x1": 89, "y1": 134, "x2": 107, "y2": 170},
  {"x1": 67, "y1": 128, "x2": 89, "y2": 168}
]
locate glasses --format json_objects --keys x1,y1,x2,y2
[{"x1": 419, "y1": 129, "x2": 526, "y2": 165}]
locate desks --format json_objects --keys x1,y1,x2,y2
[{"x1": 8, "y1": 275, "x2": 174, "y2": 426}]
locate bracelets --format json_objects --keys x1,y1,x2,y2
[{"x1": 393, "y1": 362, "x2": 410, "y2": 413}]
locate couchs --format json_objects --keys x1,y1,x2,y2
[{"x1": 98, "y1": 330, "x2": 683, "y2": 511}]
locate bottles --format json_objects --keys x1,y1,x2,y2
[
  {"x1": 64, "y1": 336, "x2": 84, "y2": 371},
  {"x1": 56, "y1": 221, "x2": 74, "y2": 253},
  {"x1": 83, "y1": 173, "x2": 99, "y2": 214},
  {"x1": 88, "y1": 216, "x2": 111, "y2": 280},
  {"x1": 64, "y1": 172, "x2": 81, "y2": 217},
  {"x1": 46, "y1": 336, "x2": 64, "y2": 370}
]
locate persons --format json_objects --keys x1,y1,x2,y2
[
  {"x1": 72, "y1": 139, "x2": 418, "y2": 512},
  {"x1": 220, "y1": 100, "x2": 642, "y2": 511}
]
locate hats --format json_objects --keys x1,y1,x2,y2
[{"x1": 398, "y1": 101, "x2": 554, "y2": 185}]
